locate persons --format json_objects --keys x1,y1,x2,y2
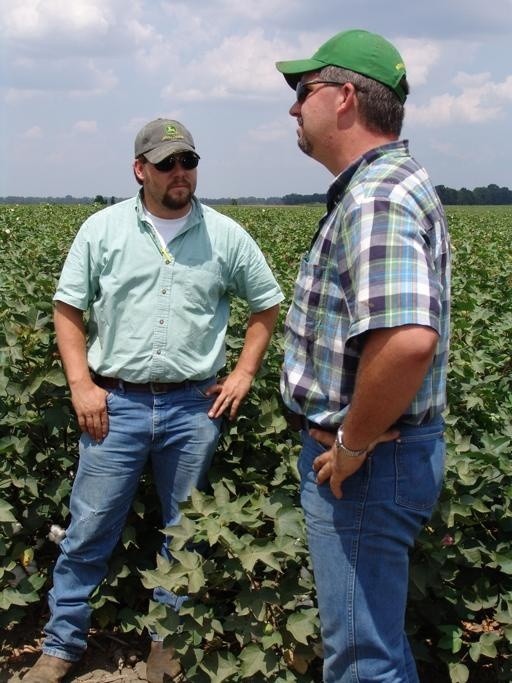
[
  {"x1": 277, "y1": 28, "x2": 453, "y2": 683},
  {"x1": 22, "y1": 117, "x2": 286, "y2": 683}
]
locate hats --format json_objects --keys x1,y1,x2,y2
[
  {"x1": 135, "y1": 117, "x2": 200, "y2": 167},
  {"x1": 274, "y1": 28, "x2": 406, "y2": 106}
]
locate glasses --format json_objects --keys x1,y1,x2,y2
[
  {"x1": 140, "y1": 152, "x2": 200, "y2": 172},
  {"x1": 294, "y1": 78, "x2": 368, "y2": 105}
]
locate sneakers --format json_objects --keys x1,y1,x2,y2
[
  {"x1": 145, "y1": 636, "x2": 204, "y2": 683},
  {"x1": 19, "y1": 653, "x2": 75, "y2": 683}
]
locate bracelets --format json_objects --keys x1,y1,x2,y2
[{"x1": 333, "y1": 424, "x2": 371, "y2": 458}]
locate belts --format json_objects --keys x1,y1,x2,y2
[{"x1": 93, "y1": 371, "x2": 208, "y2": 395}]
